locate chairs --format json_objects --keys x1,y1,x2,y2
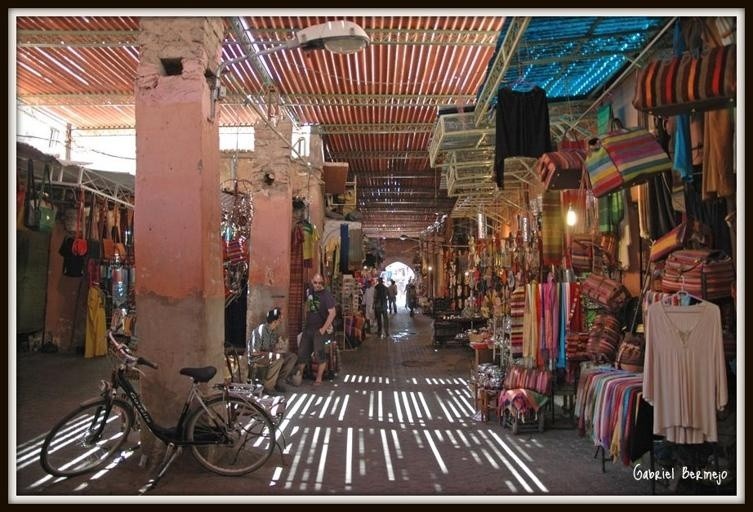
[{"x1": 247, "y1": 338, "x2": 268, "y2": 384}]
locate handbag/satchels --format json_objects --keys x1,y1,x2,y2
[
  {"x1": 662, "y1": 251, "x2": 732, "y2": 301},
  {"x1": 72, "y1": 238, "x2": 126, "y2": 261},
  {"x1": 587, "y1": 315, "x2": 621, "y2": 362},
  {"x1": 584, "y1": 127, "x2": 673, "y2": 198},
  {"x1": 650, "y1": 221, "x2": 713, "y2": 261},
  {"x1": 536, "y1": 150, "x2": 588, "y2": 192},
  {"x1": 503, "y1": 365, "x2": 551, "y2": 395},
  {"x1": 26, "y1": 188, "x2": 58, "y2": 231},
  {"x1": 222, "y1": 235, "x2": 250, "y2": 265},
  {"x1": 632, "y1": 44, "x2": 736, "y2": 117},
  {"x1": 566, "y1": 333, "x2": 588, "y2": 360},
  {"x1": 321, "y1": 322, "x2": 336, "y2": 344},
  {"x1": 461, "y1": 246, "x2": 515, "y2": 319},
  {"x1": 476, "y1": 363, "x2": 504, "y2": 389},
  {"x1": 580, "y1": 274, "x2": 632, "y2": 314},
  {"x1": 616, "y1": 332, "x2": 645, "y2": 373}
]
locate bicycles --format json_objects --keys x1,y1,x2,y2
[{"x1": 40, "y1": 324, "x2": 275, "y2": 477}]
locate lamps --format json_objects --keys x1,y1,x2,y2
[{"x1": 210, "y1": 20, "x2": 371, "y2": 118}]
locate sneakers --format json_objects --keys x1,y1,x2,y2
[
  {"x1": 274, "y1": 382, "x2": 287, "y2": 393},
  {"x1": 263, "y1": 388, "x2": 278, "y2": 396}
]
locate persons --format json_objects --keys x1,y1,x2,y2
[
  {"x1": 362, "y1": 278, "x2": 417, "y2": 339},
  {"x1": 297, "y1": 273, "x2": 337, "y2": 386},
  {"x1": 85, "y1": 282, "x2": 108, "y2": 358},
  {"x1": 248, "y1": 309, "x2": 297, "y2": 394}
]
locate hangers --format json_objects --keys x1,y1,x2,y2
[{"x1": 661, "y1": 276, "x2": 704, "y2": 306}]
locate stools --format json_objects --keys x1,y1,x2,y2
[{"x1": 468, "y1": 385, "x2": 576, "y2": 435}]
